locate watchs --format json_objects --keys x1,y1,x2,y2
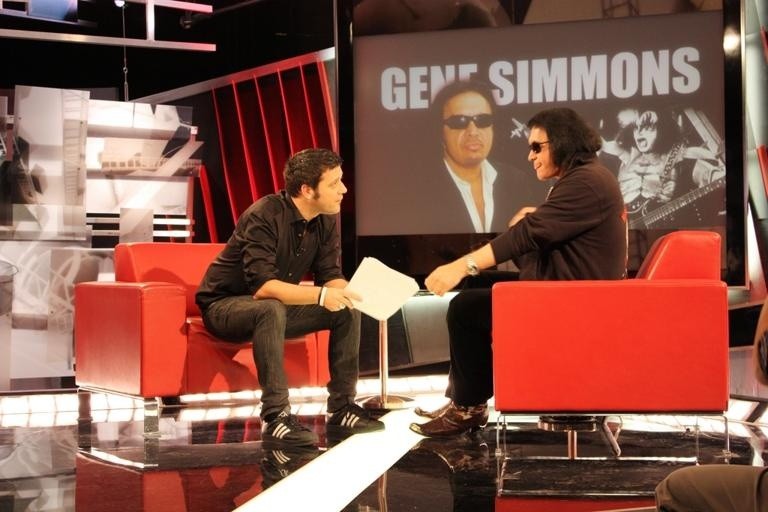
[{"x1": 463, "y1": 253, "x2": 480, "y2": 278}]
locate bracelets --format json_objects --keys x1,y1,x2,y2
[{"x1": 318, "y1": 285, "x2": 328, "y2": 307}]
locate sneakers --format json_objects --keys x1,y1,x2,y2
[
  {"x1": 260, "y1": 406, "x2": 319, "y2": 447},
  {"x1": 326, "y1": 404, "x2": 386, "y2": 434}
]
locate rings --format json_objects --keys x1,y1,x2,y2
[{"x1": 339, "y1": 302, "x2": 346, "y2": 310}]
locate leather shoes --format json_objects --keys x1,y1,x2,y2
[{"x1": 411, "y1": 399, "x2": 490, "y2": 437}]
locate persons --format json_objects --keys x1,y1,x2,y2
[
  {"x1": 406, "y1": 106, "x2": 629, "y2": 442},
  {"x1": 650, "y1": 294, "x2": 768, "y2": 510},
  {"x1": 193, "y1": 147, "x2": 387, "y2": 448},
  {"x1": 598, "y1": 104, "x2": 717, "y2": 221},
  {"x1": 399, "y1": 77, "x2": 548, "y2": 235}
]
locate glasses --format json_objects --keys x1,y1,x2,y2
[
  {"x1": 443, "y1": 112, "x2": 493, "y2": 131},
  {"x1": 528, "y1": 139, "x2": 554, "y2": 152}
]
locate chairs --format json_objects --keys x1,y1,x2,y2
[
  {"x1": 496, "y1": 456, "x2": 700, "y2": 512},
  {"x1": 75, "y1": 442, "x2": 291, "y2": 512},
  {"x1": 487, "y1": 229, "x2": 732, "y2": 454},
  {"x1": 74, "y1": 243, "x2": 329, "y2": 417}
]
